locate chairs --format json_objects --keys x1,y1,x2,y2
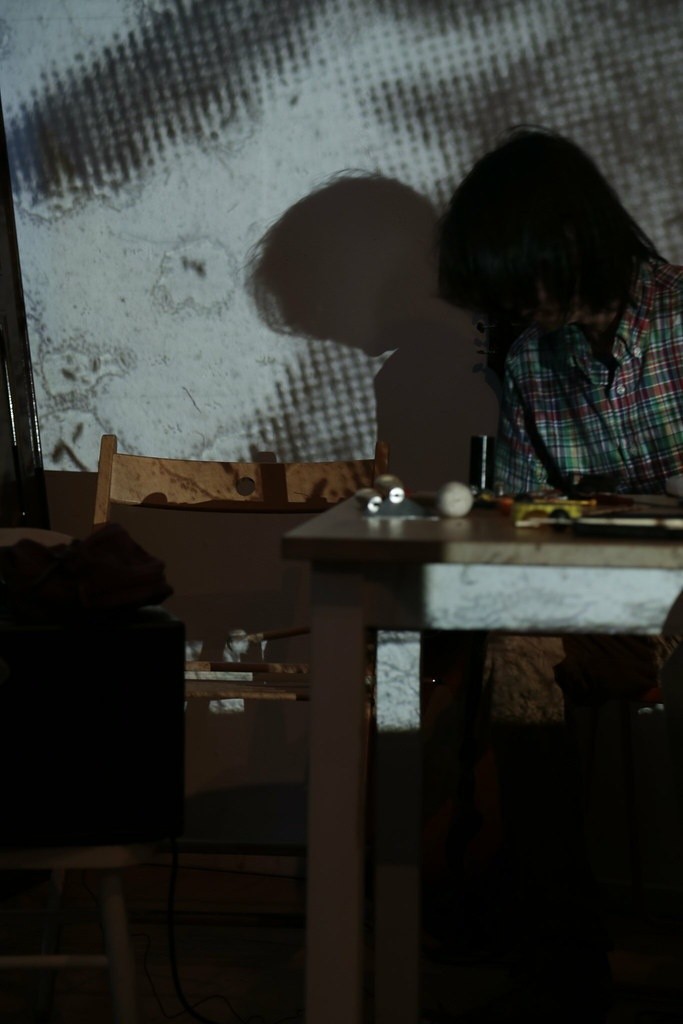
[
  {"x1": 1, "y1": 526, "x2": 161, "y2": 1024},
  {"x1": 89, "y1": 435, "x2": 395, "y2": 1023}
]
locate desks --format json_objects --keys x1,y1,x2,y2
[{"x1": 279, "y1": 486, "x2": 683, "y2": 1024}]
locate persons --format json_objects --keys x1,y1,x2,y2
[{"x1": 423, "y1": 129, "x2": 683, "y2": 872}]
